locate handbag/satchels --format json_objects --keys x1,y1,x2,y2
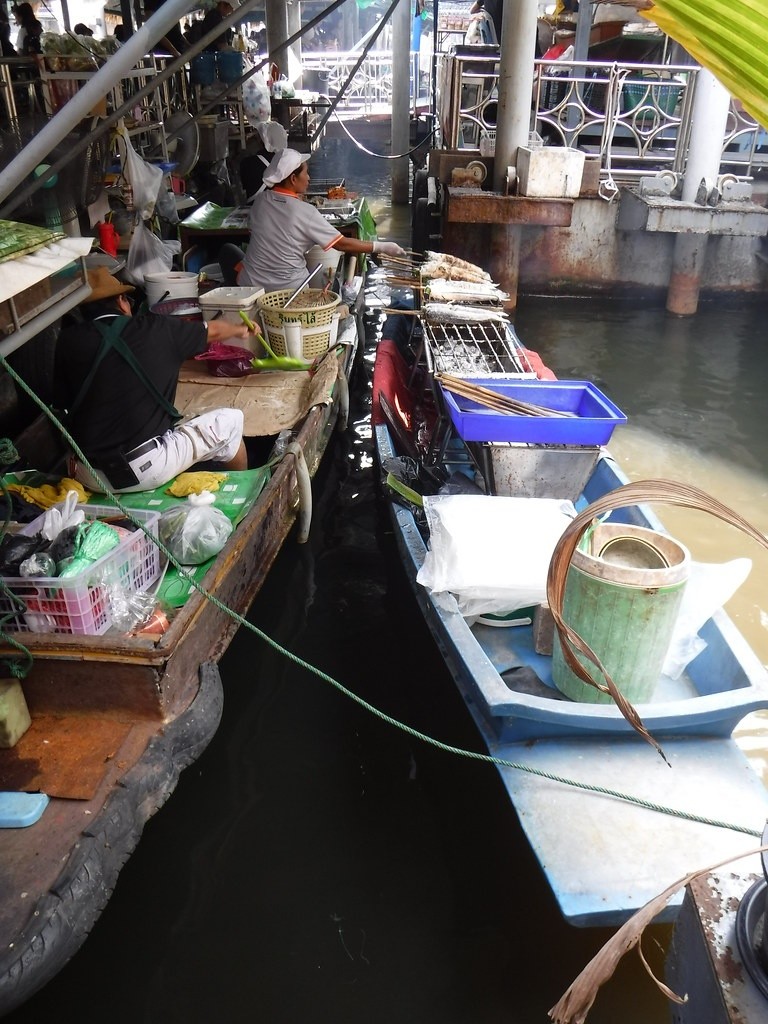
[
  {"x1": 240, "y1": 58, "x2": 271, "y2": 128},
  {"x1": 121, "y1": 127, "x2": 162, "y2": 218},
  {"x1": 126, "y1": 222, "x2": 172, "y2": 287}
]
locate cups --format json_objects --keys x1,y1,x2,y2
[
  {"x1": 274, "y1": 82, "x2": 282, "y2": 99},
  {"x1": 98, "y1": 223, "x2": 120, "y2": 260}
]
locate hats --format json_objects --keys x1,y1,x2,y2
[
  {"x1": 263, "y1": 148, "x2": 311, "y2": 188},
  {"x1": 78, "y1": 266, "x2": 136, "y2": 303}
]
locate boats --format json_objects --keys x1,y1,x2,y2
[
  {"x1": 354, "y1": 286, "x2": 768, "y2": 930},
  {"x1": 2, "y1": 218, "x2": 384, "y2": 1015}
]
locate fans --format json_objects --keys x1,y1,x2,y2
[{"x1": 145, "y1": 112, "x2": 203, "y2": 178}]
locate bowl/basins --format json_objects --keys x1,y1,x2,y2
[
  {"x1": 596, "y1": 535, "x2": 670, "y2": 569},
  {"x1": 196, "y1": 114, "x2": 219, "y2": 124}
]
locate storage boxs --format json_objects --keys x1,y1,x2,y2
[
  {"x1": 200, "y1": 122, "x2": 230, "y2": 161},
  {"x1": 0, "y1": 503, "x2": 161, "y2": 640},
  {"x1": 516, "y1": 144, "x2": 586, "y2": 198},
  {"x1": 434, "y1": 373, "x2": 628, "y2": 446}
]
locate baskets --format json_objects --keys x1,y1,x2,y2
[
  {"x1": 258, "y1": 289, "x2": 341, "y2": 359},
  {"x1": 0, "y1": 501, "x2": 162, "y2": 636}
]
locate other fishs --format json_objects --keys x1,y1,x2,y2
[{"x1": 418, "y1": 251, "x2": 510, "y2": 324}]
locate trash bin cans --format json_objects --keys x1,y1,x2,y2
[{"x1": 304, "y1": 68, "x2": 330, "y2": 119}]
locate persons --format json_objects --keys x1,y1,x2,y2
[
  {"x1": 10, "y1": 2, "x2": 45, "y2": 54},
  {"x1": 218, "y1": 148, "x2": 408, "y2": 292},
  {"x1": 72, "y1": 23, "x2": 129, "y2": 41},
  {"x1": 52, "y1": 267, "x2": 261, "y2": 493},
  {"x1": 143, "y1": 0, "x2": 244, "y2": 58}
]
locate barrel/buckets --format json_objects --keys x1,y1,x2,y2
[
  {"x1": 198, "y1": 288, "x2": 266, "y2": 359},
  {"x1": 189, "y1": 50, "x2": 244, "y2": 85},
  {"x1": 199, "y1": 262, "x2": 224, "y2": 283},
  {"x1": 304, "y1": 244, "x2": 340, "y2": 290},
  {"x1": 144, "y1": 270, "x2": 198, "y2": 306},
  {"x1": 551, "y1": 521, "x2": 691, "y2": 705}
]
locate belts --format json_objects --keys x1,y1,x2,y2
[{"x1": 73, "y1": 437, "x2": 163, "y2": 470}]
